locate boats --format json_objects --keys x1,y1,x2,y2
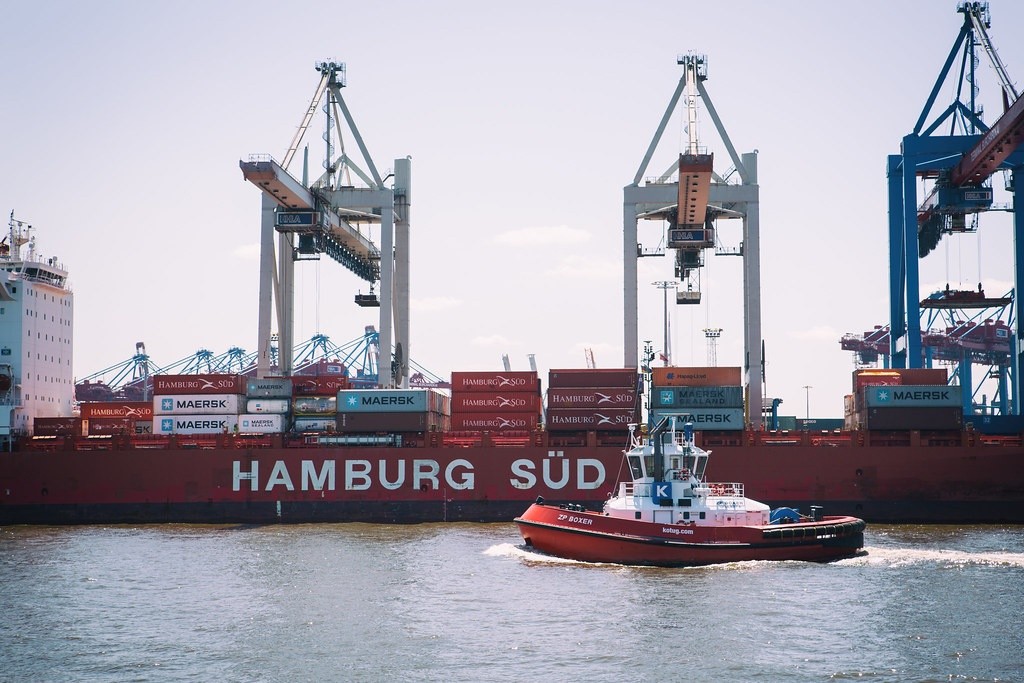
[{"x1": 513, "y1": 340, "x2": 868, "y2": 569}]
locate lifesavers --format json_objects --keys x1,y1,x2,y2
[
  {"x1": 715, "y1": 484, "x2": 726, "y2": 494},
  {"x1": 676, "y1": 520, "x2": 687, "y2": 525},
  {"x1": 679, "y1": 469, "x2": 689, "y2": 481},
  {"x1": 708, "y1": 484, "x2": 716, "y2": 493}
]
